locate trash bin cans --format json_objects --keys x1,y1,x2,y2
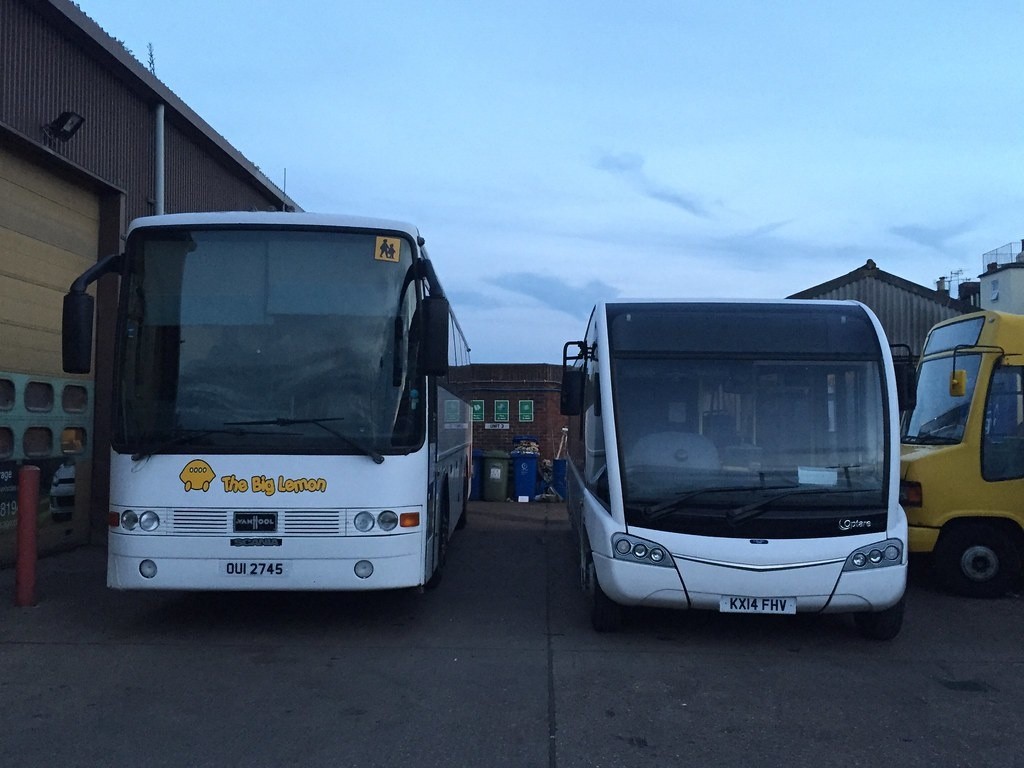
[
  {"x1": 469, "y1": 449, "x2": 483, "y2": 501},
  {"x1": 484, "y1": 451, "x2": 510, "y2": 502},
  {"x1": 554, "y1": 458, "x2": 567, "y2": 500},
  {"x1": 511, "y1": 436, "x2": 542, "y2": 503}
]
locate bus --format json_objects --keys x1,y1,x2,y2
[
  {"x1": 894, "y1": 308, "x2": 1024, "y2": 599},
  {"x1": 559, "y1": 302, "x2": 909, "y2": 632},
  {"x1": 63, "y1": 213, "x2": 474, "y2": 592}
]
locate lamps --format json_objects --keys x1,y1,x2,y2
[{"x1": 41, "y1": 111, "x2": 86, "y2": 142}]
